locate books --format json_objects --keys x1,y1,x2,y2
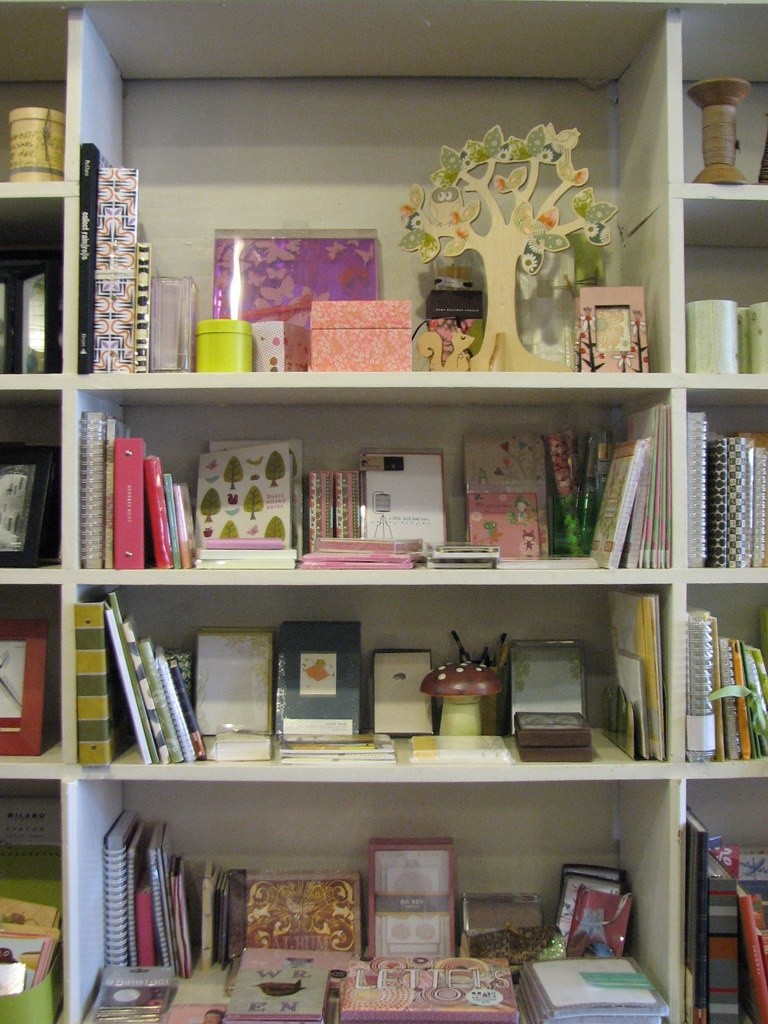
[
  {"x1": 76, "y1": 592, "x2": 665, "y2": 765},
  {"x1": 687, "y1": 411, "x2": 768, "y2": 568},
  {"x1": 686, "y1": 608, "x2": 768, "y2": 762},
  {"x1": 78, "y1": 143, "x2": 152, "y2": 375},
  {"x1": 78, "y1": 404, "x2": 671, "y2": 571},
  {"x1": 684, "y1": 804, "x2": 768, "y2": 1024},
  {"x1": 94, "y1": 809, "x2": 672, "y2": 1024},
  {"x1": 0, "y1": 898, "x2": 59, "y2": 989}
]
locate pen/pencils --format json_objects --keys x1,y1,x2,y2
[
  {"x1": 286, "y1": 743, "x2": 384, "y2": 751},
  {"x1": 450, "y1": 629, "x2": 507, "y2": 666}
]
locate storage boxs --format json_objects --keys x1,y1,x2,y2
[
  {"x1": 309, "y1": 300, "x2": 414, "y2": 371},
  {"x1": 246, "y1": 869, "x2": 361, "y2": 959},
  {"x1": 251, "y1": 322, "x2": 310, "y2": 373},
  {"x1": 369, "y1": 838, "x2": 456, "y2": 957}
]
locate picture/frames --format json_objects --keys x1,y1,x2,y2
[
  {"x1": 0, "y1": 441, "x2": 61, "y2": 567},
  {"x1": 0, "y1": 260, "x2": 63, "y2": 374},
  {"x1": 0, "y1": 619, "x2": 49, "y2": 757}
]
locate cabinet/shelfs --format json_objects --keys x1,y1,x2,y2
[{"x1": 0, "y1": 1, "x2": 768, "y2": 1024}]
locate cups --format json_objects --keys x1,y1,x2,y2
[
  {"x1": 737, "y1": 308, "x2": 752, "y2": 374},
  {"x1": 687, "y1": 299, "x2": 737, "y2": 374},
  {"x1": 751, "y1": 303, "x2": 768, "y2": 374}
]
